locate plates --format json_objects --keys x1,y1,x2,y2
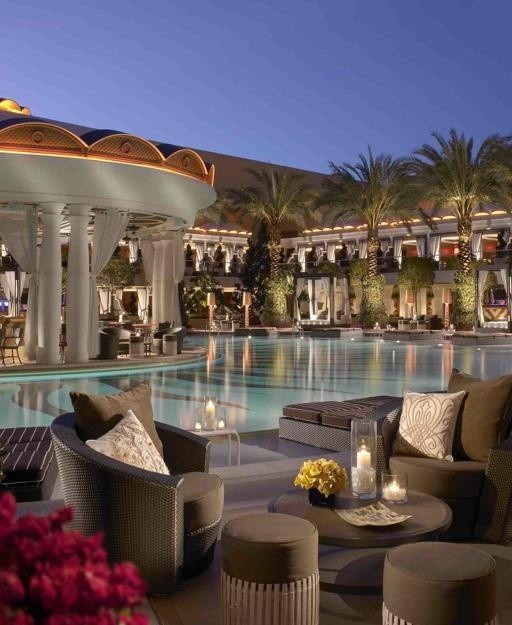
[{"x1": 333, "y1": 502, "x2": 414, "y2": 528}]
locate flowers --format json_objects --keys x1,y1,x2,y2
[
  {"x1": 293, "y1": 457, "x2": 349, "y2": 500},
  {"x1": 1, "y1": 492, "x2": 146, "y2": 625}
]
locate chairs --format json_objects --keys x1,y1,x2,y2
[
  {"x1": 383, "y1": 312, "x2": 442, "y2": 330},
  {"x1": 359, "y1": 388, "x2": 510, "y2": 544},
  {"x1": 0, "y1": 301, "x2": 186, "y2": 375},
  {"x1": 52, "y1": 411, "x2": 227, "y2": 602}
]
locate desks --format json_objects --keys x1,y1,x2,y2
[{"x1": 185, "y1": 428, "x2": 240, "y2": 468}]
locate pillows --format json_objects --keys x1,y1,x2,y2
[
  {"x1": 84, "y1": 410, "x2": 173, "y2": 474},
  {"x1": 67, "y1": 381, "x2": 165, "y2": 454},
  {"x1": 445, "y1": 368, "x2": 510, "y2": 463},
  {"x1": 398, "y1": 387, "x2": 457, "y2": 464}
]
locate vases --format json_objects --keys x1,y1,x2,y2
[{"x1": 306, "y1": 489, "x2": 338, "y2": 507}]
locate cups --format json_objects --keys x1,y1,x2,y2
[
  {"x1": 204, "y1": 396, "x2": 218, "y2": 431},
  {"x1": 218, "y1": 419, "x2": 226, "y2": 430},
  {"x1": 350, "y1": 419, "x2": 378, "y2": 500},
  {"x1": 380, "y1": 469, "x2": 408, "y2": 505},
  {"x1": 194, "y1": 422, "x2": 201, "y2": 432}
]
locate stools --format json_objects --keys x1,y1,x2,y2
[
  {"x1": 2, "y1": 423, "x2": 58, "y2": 500},
  {"x1": 221, "y1": 513, "x2": 320, "y2": 625},
  {"x1": 377, "y1": 544, "x2": 497, "y2": 625}
]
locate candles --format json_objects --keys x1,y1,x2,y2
[
  {"x1": 383, "y1": 487, "x2": 406, "y2": 500},
  {"x1": 356, "y1": 448, "x2": 372, "y2": 469},
  {"x1": 192, "y1": 399, "x2": 225, "y2": 431}
]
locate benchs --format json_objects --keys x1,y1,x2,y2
[{"x1": 278, "y1": 394, "x2": 394, "y2": 452}]
